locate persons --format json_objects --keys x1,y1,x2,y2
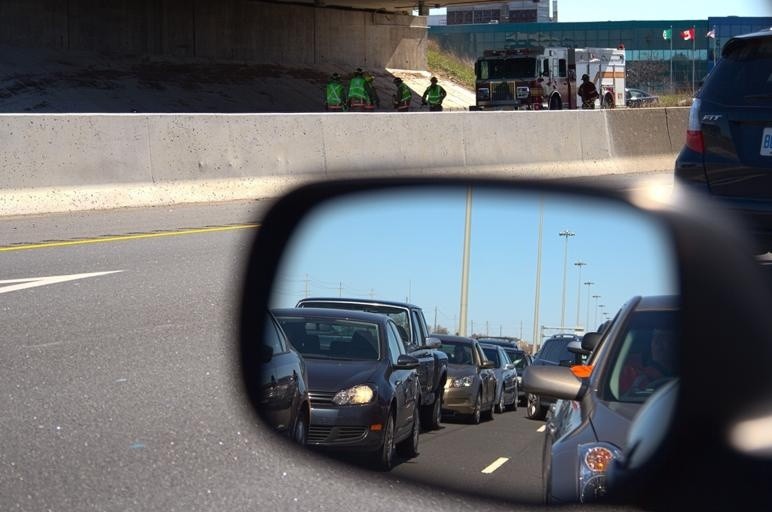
[
  {"x1": 325, "y1": 67, "x2": 380, "y2": 112},
  {"x1": 577, "y1": 74, "x2": 598, "y2": 109},
  {"x1": 453, "y1": 345, "x2": 465, "y2": 363},
  {"x1": 422, "y1": 76, "x2": 446, "y2": 112},
  {"x1": 392, "y1": 77, "x2": 412, "y2": 112}
]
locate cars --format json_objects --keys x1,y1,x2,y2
[{"x1": 624, "y1": 86, "x2": 661, "y2": 105}]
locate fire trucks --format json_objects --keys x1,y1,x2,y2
[{"x1": 468, "y1": 42, "x2": 633, "y2": 112}]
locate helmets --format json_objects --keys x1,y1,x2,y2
[
  {"x1": 353, "y1": 67, "x2": 363, "y2": 76},
  {"x1": 330, "y1": 72, "x2": 344, "y2": 81},
  {"x1": 582, "y1": 74, "x2": 589, "y2": 80},
  {"x1": 430, "y1": 77, "x2": 437, "y2": 81},
  {"x1": 394, "y1": 78, "x2": 403, "y2": 83}
]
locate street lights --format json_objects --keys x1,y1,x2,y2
[{"x1": 553, "y1": 227, "x2": 614, "y2": 340}]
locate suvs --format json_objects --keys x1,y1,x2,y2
[{"x1": 668, "y1": 22, "x2": 771, "y2": 264}]
